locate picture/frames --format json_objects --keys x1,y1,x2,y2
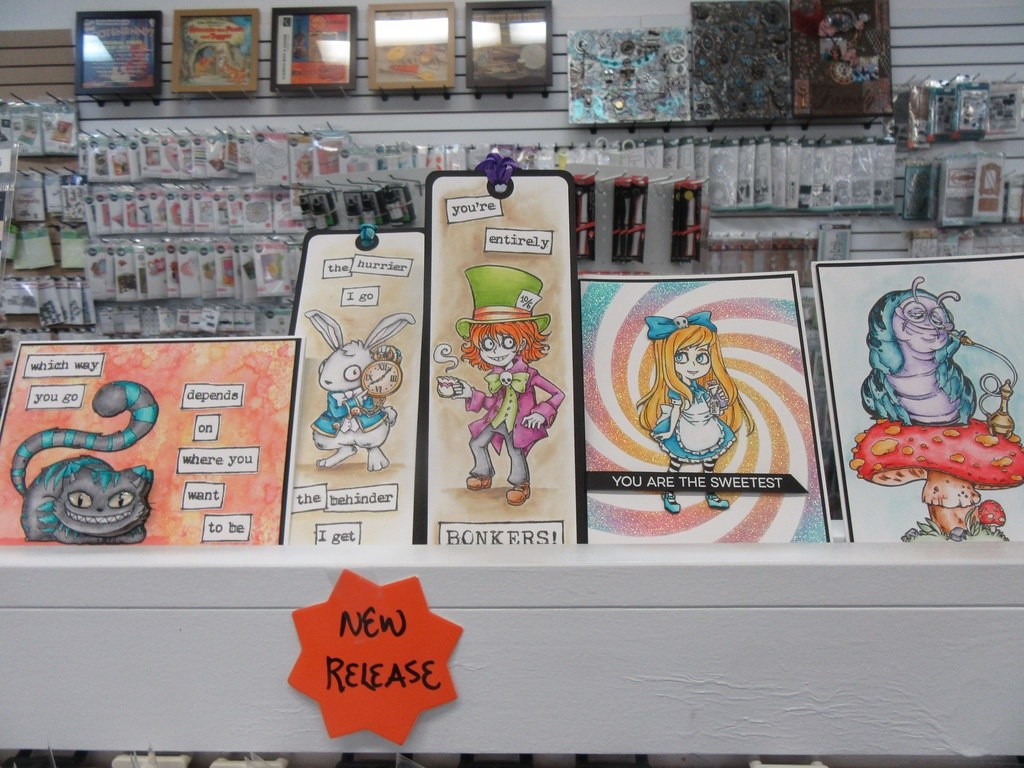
[
  {"x1": 270, "y1": 5, "x2": 361, "y2": 92},
  {"x1": 75, "y1": 10, "x2": 163, "y2": 95},
  {"x1": 465, "y1": 1, "x2": 554, "y2": 89},
  {"x1": 367, "y1": 2, "x2": 457, "y2": 91},
  {"x1": 171, "y1": 8, "x2": 261, "y2": 93}
]
